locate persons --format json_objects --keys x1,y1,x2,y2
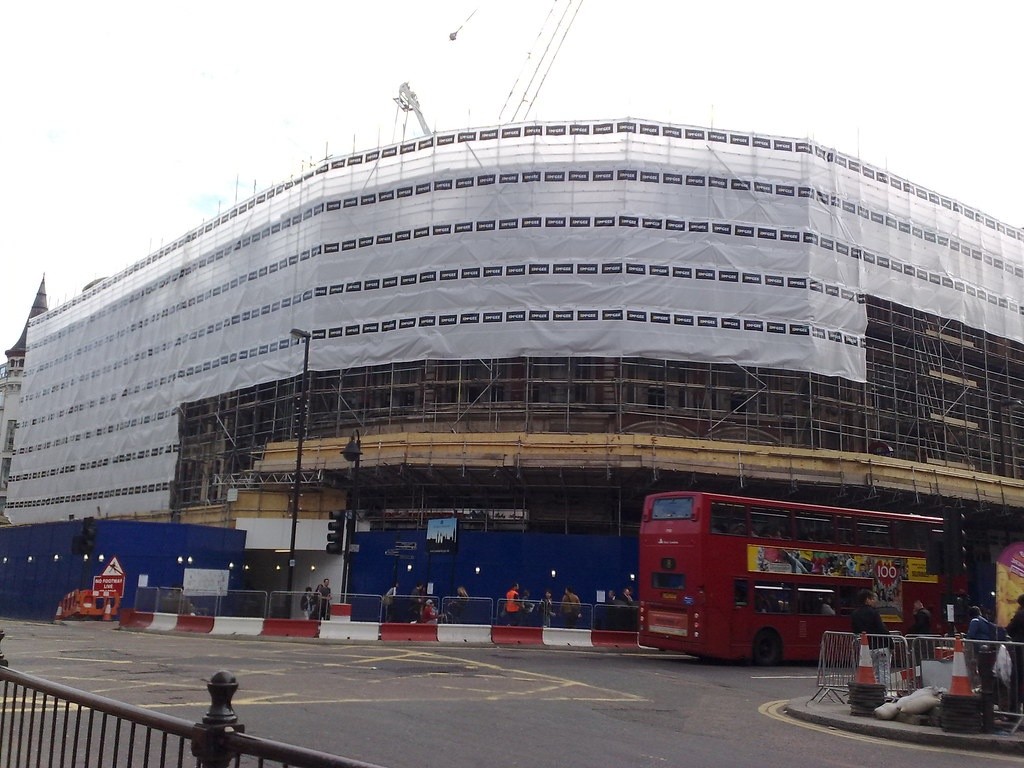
[
  {"x1": 1003, "y1": 594, "x2": 1024, "y2": 710},
  {"x1": 907, "y1": 600, "x2": 931, "y2": 634},
  {"x1": 559, "y1": 587, "x2": 580, "y2": 628},
  {"x1": 1003, "y1": 651, "x2": 1005, "y2": 652},
  {"x1": 819, "y1": 593, "x2": 838, "y2": 614},
  {"x1": 300, "y1": 579, "x2": 332, "y2": 621},
  {"x1": 608, "y1": 590, "x2": 626, "y2": 605},
  {"x1": 851, "y1": 590, "x2": 892, "y2": 650},
  {"x1": 408, "y1": 583, "x2": 468, "y2": 624},
  {"x1": 504, "y1": 583, "x2": 531, "y2": 626},
  {"x1": 967, "y1": 606, "x2": 998, "y2": 693},
  {"x1": 540, "y1": 592, "x2": 556, "y2": 628},
  {"x1": 622, "y1": 588, "x2": 633, "y2": 606}
]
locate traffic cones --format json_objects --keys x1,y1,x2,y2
[
  {"x1": 55, "y1": 601, "x2": 63, "y2": 620},
  {"x1": 949, "y1": 635, "x2": 973, "y2": 695},
  {"x1": 855, "y1": 631, "x2": 877, "y2": 684},
  {"x1": 103, "y1": 599, "x2": 112, "y2": 621}
]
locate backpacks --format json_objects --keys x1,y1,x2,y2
[{"x1": 978, "y1": 616, "x2": 1015, "y2": 661}]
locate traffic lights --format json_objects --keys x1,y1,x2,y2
[
  {"x1": 81, "y1": 524, "x2": 96, "y2": 555},
  {"x1": 944, "y1": 505, "x2": 969, "y2": 597}
]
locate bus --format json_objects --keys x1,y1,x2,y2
[{"x1": 635, "y1": 491, "x2": 973, "y2": 667}]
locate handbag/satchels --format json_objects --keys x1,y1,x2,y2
[{"x1": 572, "y1": 609, "x2": 583, "y2": 622}]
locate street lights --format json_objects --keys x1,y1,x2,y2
[
  {"x1": 284, "y1": 328, "x2": 311, "y2": 619},
  {"x1": 329, "y1": 428, "x2": 364, "y2": 624}
]
